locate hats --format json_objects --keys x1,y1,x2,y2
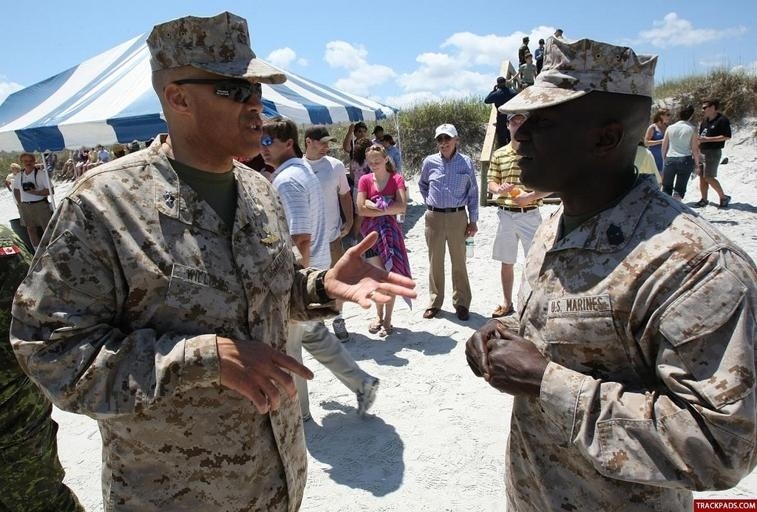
[
  {"x1": 145, "y1": 11, "x2": 286, "y2": 84},
  {"x1": 497, "y1": 36, "x2": 659, "y2": 115},
  {"x1": 304, "y1": 125, "x2": 337, "y2": 143},
  {"x1": 507, "y1": 111, "x2": 529, "y2": 121},
  {"x1": 434, "y1": 123, "x2": 458, "y2": 140}
]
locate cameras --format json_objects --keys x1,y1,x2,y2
[{"x1": 21, "y1": 181, "x2": 35, "y2": 191}]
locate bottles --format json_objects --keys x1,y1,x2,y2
[
  {"x1": 700, "y1": 128, "x2": 707, "y2": 136},
  {"x1": 509, "y1": 188, "x2": 528, "y2": 207},
  {"x1": 464, "y1": 231, "x2": 474, "y2": 258},
  {"x1": 396, "y1": 213, "x2": 405, "y2": 224}
]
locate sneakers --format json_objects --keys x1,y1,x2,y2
[
  {"x1": 719, "y1": 196, "x2": 730, "y2": 210},
  {"x1": 692, "y1": 199, "x2": 708, "y2": 208},
  {"x1": 333, "y1": 305, "x2": 515, "y2": 344},
  {"x1": 357, "y1": 377, "x2": 378, "y2": 416}
]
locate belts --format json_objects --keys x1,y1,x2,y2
[
  {"x1": 500, "y1": 205, "x2": 537, "y2": 213},
  {"x1": 427, "y1": 205, "x2": 465, "y2": 212}
]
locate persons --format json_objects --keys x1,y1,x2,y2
[
  {"x1": 645, "y1": 110, "x2": 669, "y2": 179},
  {"x1": 0, "y1": 225, "x2": 82, "y2": 512},
  {"x1": 693, "y1": 100, "x2": 731, "y2": 209},
  {"x1": 661, "y1": 104, "x2": 700, "y2": 203},
  {"x1": 487, "y1": 108, "x2": 554, "y2": 318},
  {"x1": 236, "y1": 116, "x2": 412, "y2": 425},
  {"x1": 484, "y1": 30, "x2": 569, "y2": 147},
  {"x1": 418, "y1": 123, "x2": 478, "y2": 320},
  {"x1": 634, "y1": 144, "x2": 661, "y2": 193},
  {"x1": 10, "y1": 12, "x2": 418, "y2": 512},
  {"x1": 5, "y1": 139, "x2": 152, "y2": 252},
  {"x1": 467, "y1": 38, "x2": 757, "y2": 512}
]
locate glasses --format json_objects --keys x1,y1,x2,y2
[
  {"x1": 260, "y1": 135, "x2": 279, "y2": 147},
  {"x1": 365, "y1": 147, "x2": 386, "y2": 152},
  {"x1": 434, "y1": 135, "x2": 451, "y2": 143},
  {"x1": 163, "y1": 77, "x2": 262, "y2": 105}
]
